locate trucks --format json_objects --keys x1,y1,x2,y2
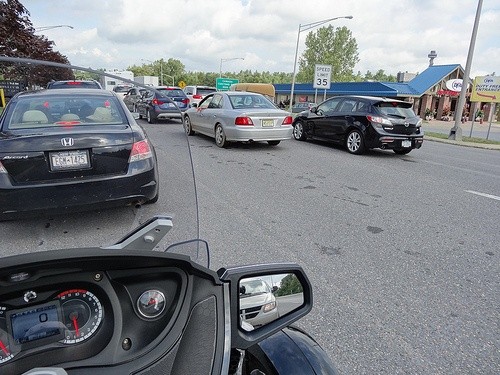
[{"x1": 227, "y1": 83, "x2": 275, "y2": 104}]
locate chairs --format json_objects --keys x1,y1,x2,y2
[{"x1": 340, "y1": 103, "x2": 353, "y2": 113}]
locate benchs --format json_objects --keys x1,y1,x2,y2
[{"x1": 22, "y1": 107, "x2": 112, "y2": 123}]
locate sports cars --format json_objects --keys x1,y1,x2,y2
[{"x1": 0, "y1": 56, "x2": 337, "y2": 375}]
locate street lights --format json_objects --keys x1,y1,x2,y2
[
  {"x1": 162, "y1": 74, "x2": 174, "y2": 87},
  {"x1": 288, "y1": 16, "x2": 354, "y2": 114},
  {"x1": 220, "y1": 57, "x2": 244, "y2": 78}
]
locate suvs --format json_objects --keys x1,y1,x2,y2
[
  {"x1": 136, "y1": 88, "x2": 190, "y2": 124},
  {"x1": 182, "y1": 86, "x2": 218, "y2": 108},
  {"x1": 123, "y1": 88, "x2": 147, "y2": 113}
]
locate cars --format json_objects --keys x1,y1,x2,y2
[
  {"x1": 47, "y1": 79, "x2": 103, "y2": 89},
  {"x1": 240, "y1": 280, "x2": 281, "y2": 330},
  {"x1": 0, "y1": 88, "x2": 160, "y2": 221},
  {"x1": 182, "y1": 92, "x2": 293, "y2": 148},
  {"x1": 288, "y1": 102, "x2": 318, "y2": 113},
  {"x1": 113, "y1": 83, "x2": 132, "y2": 92},
  {"x1": 292, "y1": 95, "x2": 423, "y2": 155}
]
